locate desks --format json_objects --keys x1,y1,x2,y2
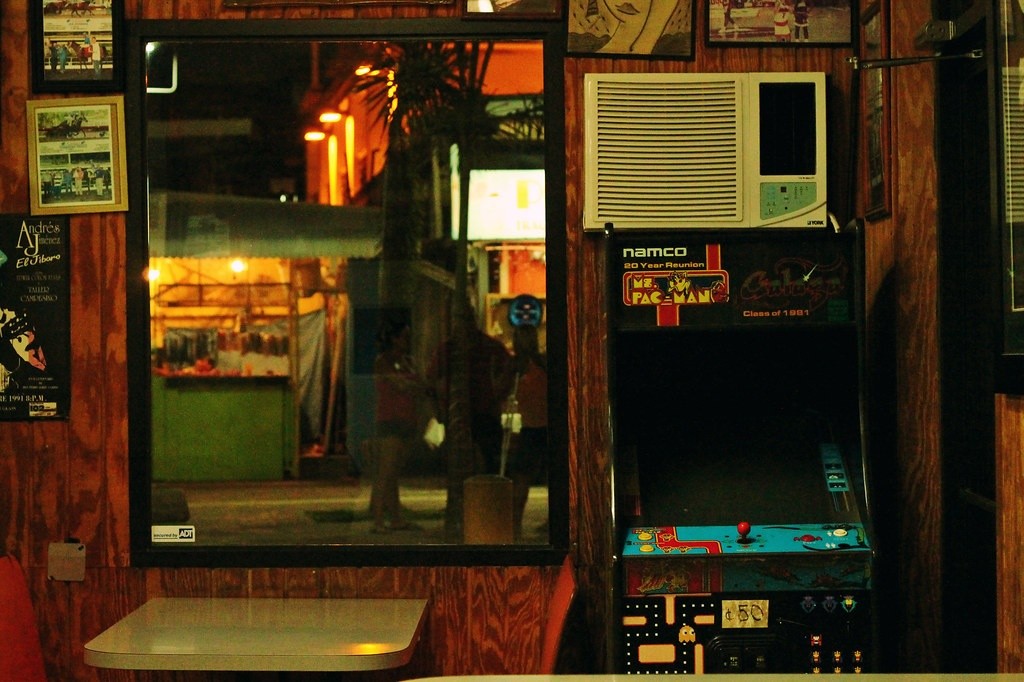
[
  {"x1": 151, "y1": 374, "x2": 295, "y2": 481},
  {"x1": 85, "y1": 598, "x2": 428, "y2": 671}
]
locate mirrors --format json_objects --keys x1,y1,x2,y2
[{"x1": 118, "y1": 19, "x2": 570, "y2": 568}]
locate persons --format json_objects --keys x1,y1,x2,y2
[{"x1": 373, "y1": 298, "x2": 548, "y2": 539}]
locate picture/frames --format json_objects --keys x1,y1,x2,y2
[
  {"x1": 26, "y1": 95, "x2": 128, "y2": 216},
  {"x1": 857, "y1": 0, "x2": 891, "y2": 224},
  {"x1": 704, "y1": 1, "x2": 857, "y2": 47},
  {"x1": 27, "y1": 0, "x2": 125, "y2": 94},
  {"x1": 562, "y1": 0, "x2": 697, "y2": 62},
  {"x1": 458, "y1": 0, "x2": 570, "y2": 20}
]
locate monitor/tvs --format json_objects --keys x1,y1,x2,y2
[{"x1": 635, "y1": 379, "x2": 836, "y2": 526}]
